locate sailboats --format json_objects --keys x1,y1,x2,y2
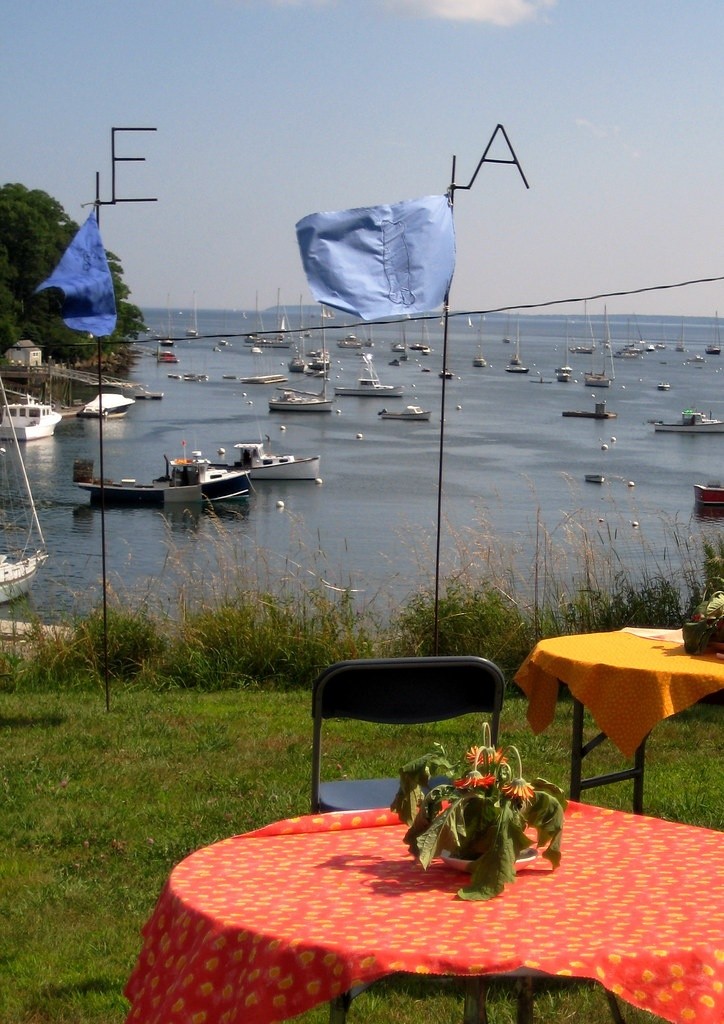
[
  {"x1": 472, "y1": 314, "x2": 486, "y2": 367},
  {"x1": 555, "y1": 319, "x2": 573, "y2": 383},
  {"x1": 705, "y1": 311, "x2": 721, "y2": 355},
  {"x1": 583, "y1": 305, "x2": 615, "y2": 387},
  {"x1": 268, "y1": 304, "x2": 334, "y2": 412},
  {"x1": 511, "y1": 312, "x2": 523, "y2": 364},
  {"x1": 676, "y1": 320, "x2": 684, "y2": 352},
  {"x1": 568, "y1": 300, "x2": 596, "y2": 354},
  {"x1": 185, "y1": 296, "x2": 199, "y2": 336},
  {"x1": 613, "y1": 312, "x2": 666, "y2": 358},
  {"x1": 244, "y1": 287, "x2": 331, "y2": 377},
  {"x1": 337, "y1": 317, "x2": 375, "y2": 348},
  {"x1": 0, "y1": 380, "x2": 49, "y2": 604},
  {"x1": 503, "y1": 310, "x2": 511, "y2": 344},
  {"x1": 388, "y1": 320, "x2": 431, "y2": 366},
  {"x1": 158, "y1": 294, "x2": 174, "y2": 346}
]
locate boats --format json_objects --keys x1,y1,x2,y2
[
  {"x1": 78, "y1": 439, "x2": 253, "y2": 509},
  {"x1": 694, "y1": 484, "x2": 724, "y2": 505},
  {"x1": 333, "y1": 352, "x2": 405, "y2": 397},
  {"x1": 77, "y1": 393, "x2": 137, "y2": 418},
  {"x1": 0, "y1": 392, "x2": 62, "y2": 441},
  {"x1": 654, "y1": 409, "x2": 724, "y2": 432},
  {"x1": 686, "y1": 354, "x2": 705, "y2": 362},
  {"x1": 159, "y1": 350, "x2": 177, "y2": 364},
  {"x1": 504, "y1": 366, "x2": 530, "y2": 373},
  {"x1": 584, "y1": 474, "x2": 605, "y2": 483},
  {"x1": 377, "y1": 406, "x2": 431, "y2": 419},
  {"x1": 439, "y1": 370, "x2": 454, "y2": 380},
  {"x1": 210, "y1": 434, "x2": 321, "y2": 481}
]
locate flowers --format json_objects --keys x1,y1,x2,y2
[
  {"x1": 390, "y1": 722, "x2": 567, "y2": 901},
  {"x1": 683, "y1": 577, "x2": 724, "y2": 654}
]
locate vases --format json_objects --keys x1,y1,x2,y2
[{"x1": 707, "y1": 618, "x2": 724, "y2": 642}]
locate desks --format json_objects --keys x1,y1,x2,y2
[
  {"x1": 513, "y1": 626, "x2": 724, "y2": 814},
  {"x1": 121, "y1": 799, "x2": 724, "y2": 1024}
]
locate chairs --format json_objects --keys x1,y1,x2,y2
[{"x1": 312, "y1": 656, "x2": 506, "y2": 814}]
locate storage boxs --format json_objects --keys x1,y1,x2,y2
[
  {"x1": 121, "y1": 479, "x2": 135, "y2": 486},
  {"x1": 73, "y1": 458, "x2": 94, "y2": 483}
]
